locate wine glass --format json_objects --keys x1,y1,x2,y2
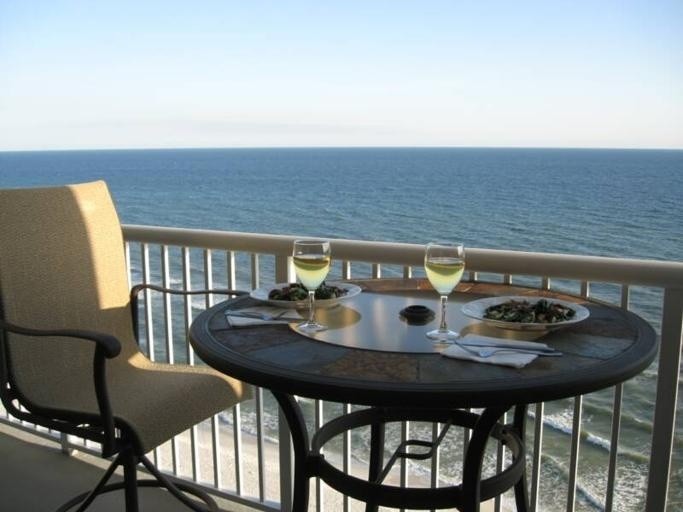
[
  {"x1": 424, "y1": 241, "x2": 466, "y2": 342},
  {"x1": 292, "y1": 239, "x2": 332, "y2": 333}
]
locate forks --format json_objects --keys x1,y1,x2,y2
[
  {"x1": 240, "y1": 309, "x2": 288, "y2": 322},
  {"x1": 454, "y1": 339, "x2": 564, "y2": 358}
]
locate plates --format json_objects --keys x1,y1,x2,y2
[
  {"x1": 248, "y1": 279, "x2": 362, "y2": 311},
  {"x1": 460, "y1": 295, "x2": 592, "y2": 331}
]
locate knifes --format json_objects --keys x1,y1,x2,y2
[
  {"x1": 225, "y1": 310, "x2": 306, "y2": 323},
  {"x1": 440, "y1": 337, "x2": 556, "y2": 352}
]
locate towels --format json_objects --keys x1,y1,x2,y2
[
  {"x1": 226, "y1": 304, "x2": 306, "y2": 328},
  {"x1": 441, "y1": 332, "x2": 547, "y2": 371}
]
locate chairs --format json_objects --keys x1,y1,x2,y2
[{"x1": 0, "y1": 179, "x2": 254, "y2": 512}]
